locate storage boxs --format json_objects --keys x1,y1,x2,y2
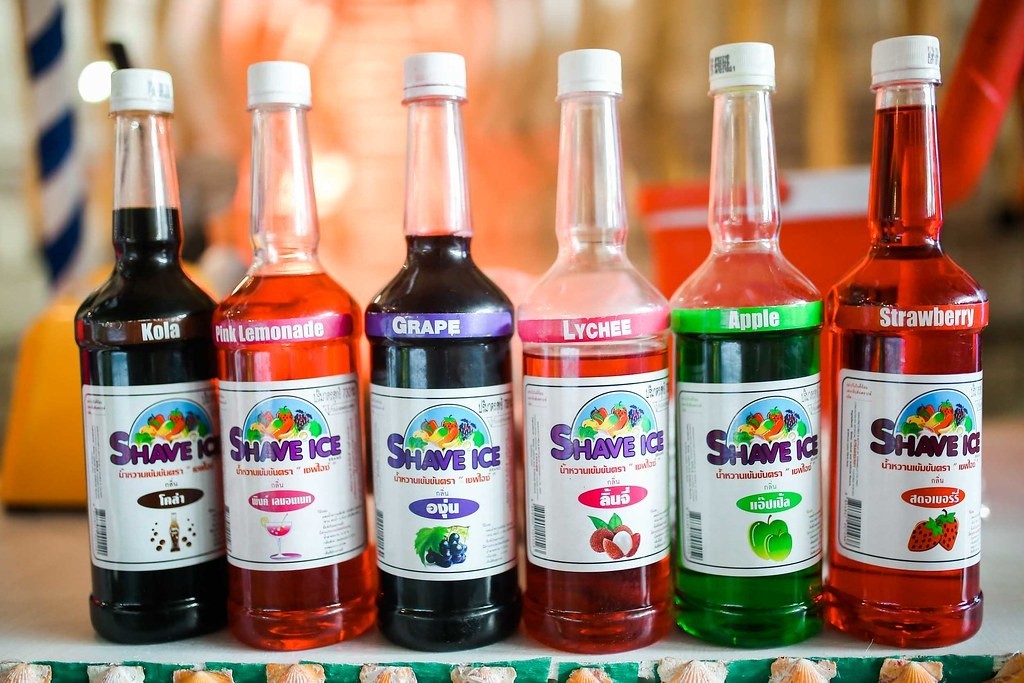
[{"x1": 635, "y1": 0, "x2": 1024, "y2": 301}]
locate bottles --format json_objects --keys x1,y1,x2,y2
[
  {"x1": 669, "y1": 43, "x2": 825, "y2": 650},
  {"x1": 212, "y1": 61, "x2": 380, "y2": 651},
  {"x1": 826, "y1": 35, "x2": 989, "y2": 649},
  {"x1": 518, "y1": 49, "x2": 673, "y2": 653},
  {"x1": 366, "y1": 52, "x2": 523, "y2": 651},
  {"x1": 74, "y1": 68, "x2": 229, "y2": 646}
]
